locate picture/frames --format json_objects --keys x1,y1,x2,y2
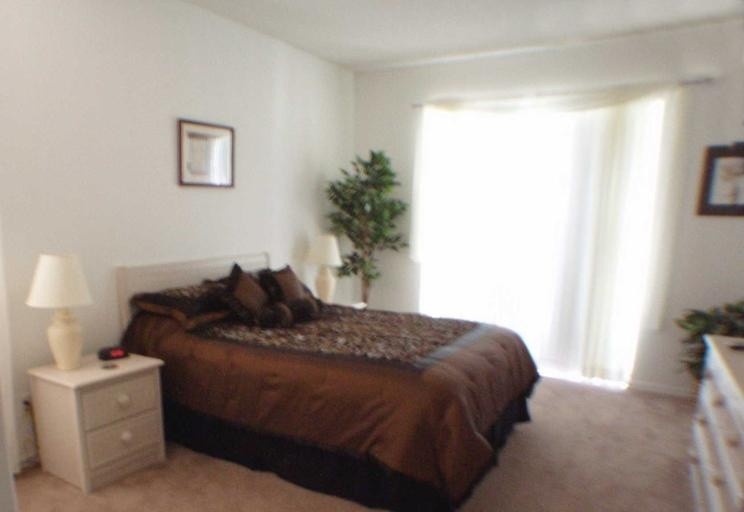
[
  {"x1": 177, "y1": 118, "x2": 236, "y2": 188},
  {"x1": 696, "y1": 145, "x2": 743, "y2": 217}
]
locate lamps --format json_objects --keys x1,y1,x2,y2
[
  {"x1": 305, "y1": 234, "x2": 344, "y2": 304},
  {"x1": 25, "y1": 253, "x2": 97, "y2": 372}
]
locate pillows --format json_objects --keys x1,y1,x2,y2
[
  {"x1": 259, "y1": 263, "x2": 311, "y2": 308},
  {"x1": 221, "y1": 262, "x2": 270, "y2": 325},
  {"x1": 130, "y1": 281, "x2": 232, "y2": 332}
]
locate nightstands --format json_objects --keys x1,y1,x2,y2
[{"x1": 24, "y1": 351, "x2": 164, "y2": 494}]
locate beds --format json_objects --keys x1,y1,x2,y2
[{"x1": 120, "y1": 304, "x2": 542, "y2": 512}]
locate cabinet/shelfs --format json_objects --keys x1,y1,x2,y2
[{"x1": 691, "y1": 335, "x2": 743, "y2": 512}]
